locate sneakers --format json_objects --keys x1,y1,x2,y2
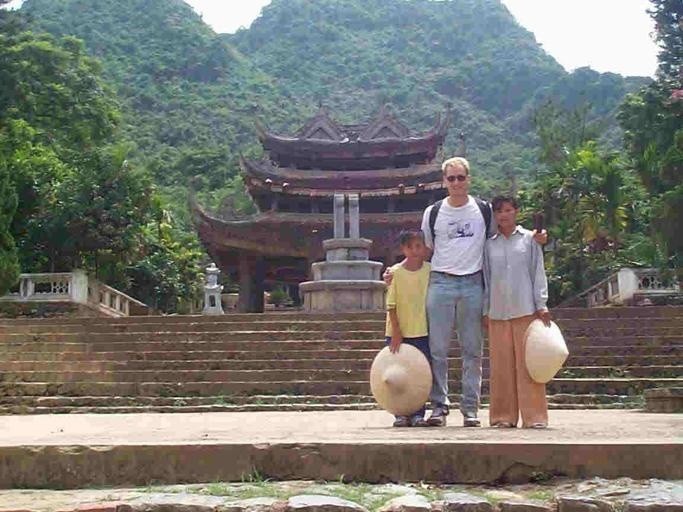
[
  {"x1": 408, "y1": 415, "x2": 429, "y2": 427},
  {"x1": 394, "y1": 416, "x2": 412, "y2": 426},
  {"x1": 428, "y1": 405, "x2": 448, "y2": 426},
  {"x1": 464, "y1": 411, "x2": 480, "y2": 426}
]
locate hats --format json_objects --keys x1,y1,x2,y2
[
  {"x1": 369, "y1": 343, "x2": 433, "y2": 416},
  {"x1": 524, "y1": 318, "x2": 569, "y2": 383}
]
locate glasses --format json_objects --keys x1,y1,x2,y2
[{"x1": 445, "y1": 174, "x2": 466, "y2": 181}]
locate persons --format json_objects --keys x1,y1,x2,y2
[
  {"x1": 380, "y1": 156, "x2": 547, "y2": 426},
  {"x1": 384, "y1": 226, "x2": 430, "y2": 426},
  {"x1": 482, "y1": 193, "x2": 552, "y2": 429}
]
